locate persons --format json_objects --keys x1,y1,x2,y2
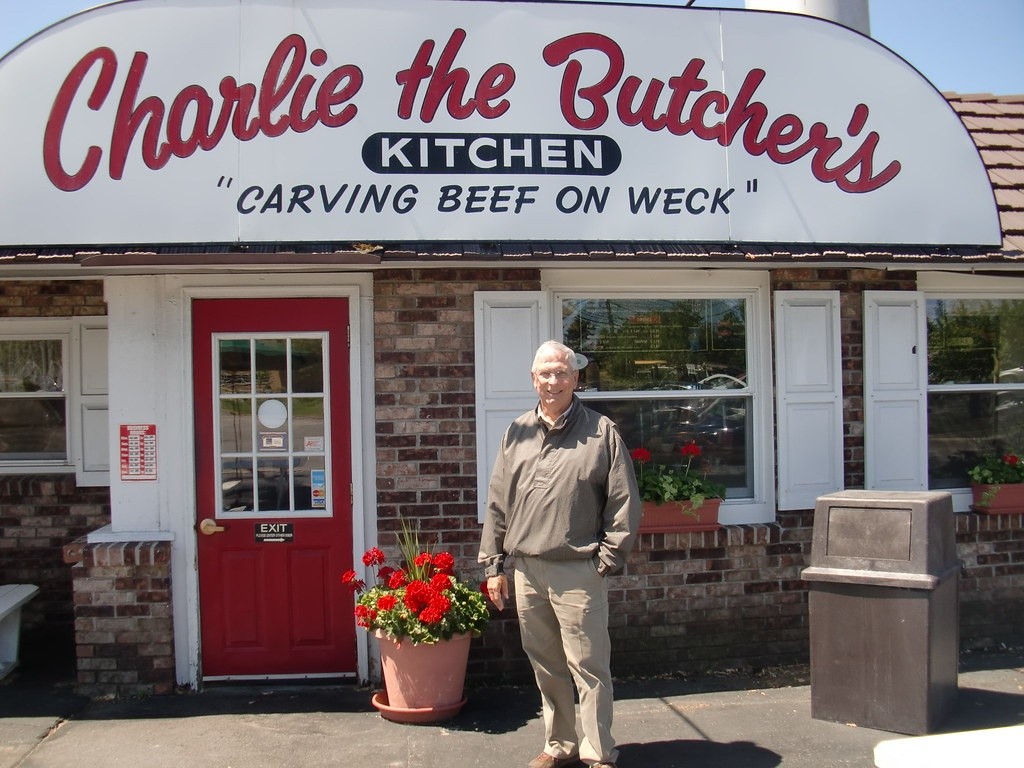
[{"x1": 475, "y1": 340, "x2": 641, "y2": 768}]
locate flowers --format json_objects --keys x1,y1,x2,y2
[
  {"x1": 340, "y1": 511, "x2": 493, "y2": 648},
  {"x1": 969, "y1": 453, "x2": 1024, "y2": 506},
  {"x1": 631, "y1": 442, "x2": 727, "y2": 520}
]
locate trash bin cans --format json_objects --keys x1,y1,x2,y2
[{"x1": 799, "y1": 489, "x2": 959, "y2": 737}]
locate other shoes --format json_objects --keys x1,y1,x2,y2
[
  {"x1": 590, "y1": 762, "x2": 618, "y2": 768},
  {"x1": 526, "y1": 752, "x2": 579, "y2": 768}
]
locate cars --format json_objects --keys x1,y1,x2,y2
[{"x1": 574, "y1": 361, "x2": 749, "y2": 470}]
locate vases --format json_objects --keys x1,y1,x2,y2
[
  {"x1": 373, "y1": 628, "x2": 474, "y2": 723},
  {"x1": 973, "y1": 484, "x2": 1024, "y2": 517},
  {"x1": 635, "y1": 499, "x2": 722, "y2": 536}
]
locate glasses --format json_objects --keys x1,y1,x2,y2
[{"x1": 533, "y1": 369, "x2": 575, "y2": 381}]
